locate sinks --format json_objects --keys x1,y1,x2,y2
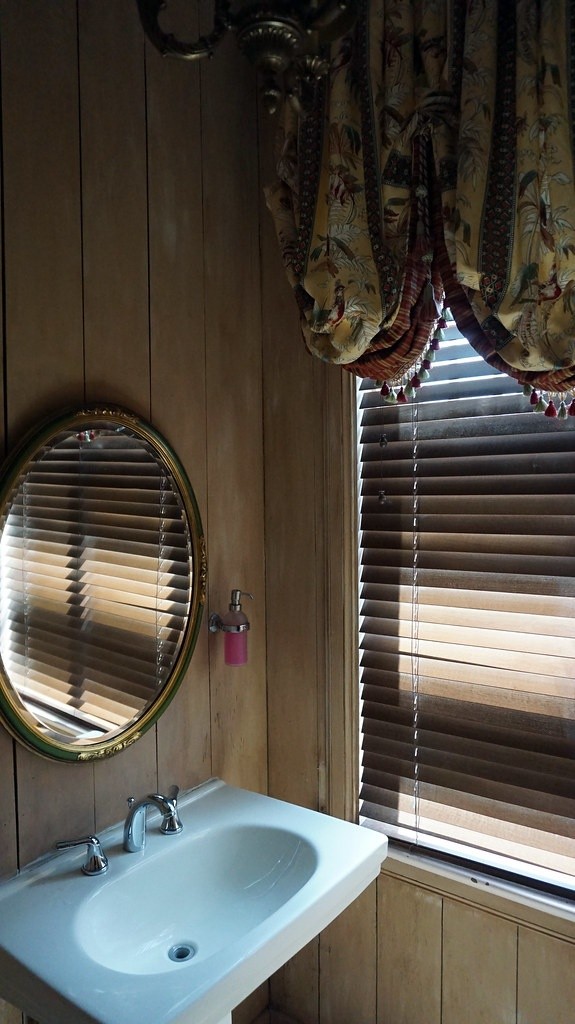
[{"x1": 72, "y1": 819, "x2": 321, "y2": 978}]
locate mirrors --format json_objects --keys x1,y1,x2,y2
[{"x1": 1, "y1": 407, "x2": 207, "y2": 765}]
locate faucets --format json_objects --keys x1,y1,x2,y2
[{"x1": 122, "y1": 792, "x2": 184, "y2": 854}]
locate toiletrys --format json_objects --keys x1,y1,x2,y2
[{"x1": 222, "y1": 588, "x2": 255, "y2": 668}]
